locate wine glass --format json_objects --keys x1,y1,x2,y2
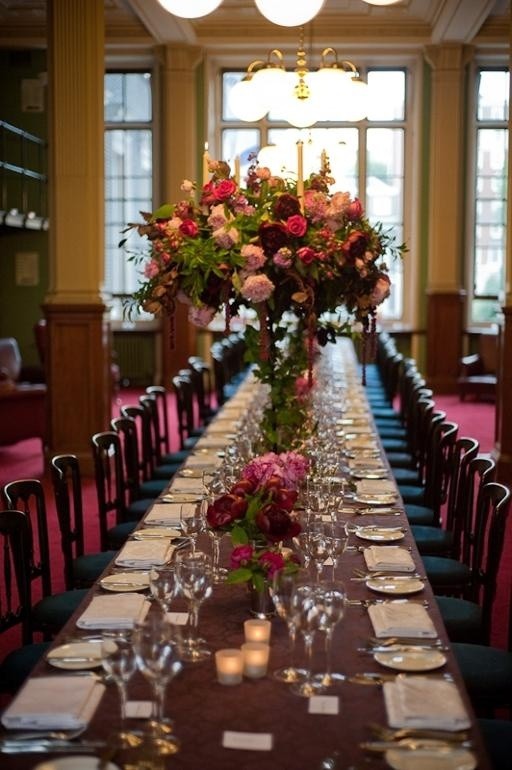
[
  {"x1": 271, "y1": 565, "x2": 313, "y2": 684},
  {"x1": 149, "y1": 385, "x2": 270, "y2": 661},
  {"x1": 290, "y1": 583, "x2": 327, "y2": 698},
  {"x1": 134, "y1": 636, "x2": 181, "y2": 757},
  {"x1": 312, "y1": 580, "x2": 346, "y2": 686},
  {"x1": 100, "y1": 628, "x2": 144, "y2": 749},
  {"x1": 298, "y1": 341, "x2": 344, "y2": 583},
  {"x1": 132, "y1": 611, "x2": 175, "y2": 736}
]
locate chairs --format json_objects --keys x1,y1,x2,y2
[{"x1": 457, "y1": 333, "x2": 498, "y2": 402}]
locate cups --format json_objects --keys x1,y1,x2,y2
[
  {"x1": 240, "y1": 642, "x2": 270, "y2": 680},
  {"x1": 215, "y1": 649, "x2": 244, "y2": 689}
]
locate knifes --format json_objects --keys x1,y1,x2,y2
[
  {"x1": 99, "y1": 582, "x2": 150, "y2": 587},
  {"x1": 43, "y1": 655, "x2": 114, "y2": 663},
  {"x1": 1, "y1": 738, "x2": 107, "y2": 755},
  {"x1": 357, "y1": 647, "x2": 450, "y2": 654},
  {"x1": 352, "y1": 576, "x2": 428, "y2": 581},
  {"x1": 359, "y1": 740, "x2": 473, "y2": 752}
]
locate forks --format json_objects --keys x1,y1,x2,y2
[
  {"x1": 350, "y1": 569, "x2": 418, "y2": 577},
  {"x1": 369, "y1": 721, "x2": 468, "y2": 743},
  {"x1": 8, "y1": 724, "x2": 85, "y2": 740},
  {"x1": 357, "y1": 636, "x2": 449, "y2": 647},
  {"x1": 349, "y1": 598, "x2": 432, "y2": 611}
]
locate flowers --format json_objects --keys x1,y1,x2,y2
[{"x1": 118, "y1": 149, "x2": 409, "y2": 452}]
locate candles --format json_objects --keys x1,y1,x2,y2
[
  {"x1": 202, "y1": 140, "x2": 210, "y2": 187},
  {"x1": 296, "y1": 139, "x2": 303, "y2": 196},
  {"x1": 321, "y1": 148, "x2": 327, "y2": 176},
  {"x1": 234, "y1": 152, "x2": 241, "y2": 185}
]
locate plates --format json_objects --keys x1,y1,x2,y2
[
  {"x1": 366, "y1": 576, "x2": 424, "y2": 594},
  {"x1": 356, "y1": 527, "x2": 405, "y2": 541},
  {"x1": 335, "y1": 340, "x2": 400, "y2": 505},
  {"x1": 132, "y1": 364, "x2": 260, "y2": 542},
  {"x1": 101, "y1": 573, "x2": 159, "y2": 592},
  {"x1": 385, "y1": 738, "x2": 478, "y2": 770},
  {"x1": 374, "y1": 644, "x2": 447, "y2": 671},
  {"x1": 46, "y1": 641, "x2": 118, "y2": 670},
  {"x1": 36, "y1": 756, "x2": 120, "y2": 769}
]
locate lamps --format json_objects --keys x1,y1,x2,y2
[
  {"x1": 257, "y1": 129, "x2": 353, "y2": 182},
  {"x1": 158, "y1": 0, "x2": 399, "y2": 27},
  {"x1": 228, "y1": 26, "x2": 376, "y2": 128}
]
